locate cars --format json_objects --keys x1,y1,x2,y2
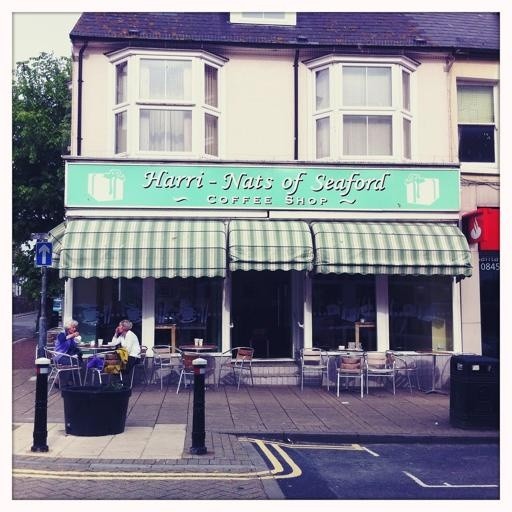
[
  {"x1": 314, "y1": 315, "x2": 352, "y2": 336},
  {"x1": 340, "y1": 311, "x2": 452, "y2": 351},
  {"x1": 53, "y1": 297, "x2": 63, "y2": 320}
]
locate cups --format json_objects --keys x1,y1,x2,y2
[
  {"x1": 198, "y1": 338, "x2": 204, "y2": 347},
  {"x1": 194, "y1": 338, "x2": 199, "y2": 347},
  {"x1": 73, "y1": 333, "x2": 82, "y2": 343},
  {"x1": 90, "y1": 340, "x2": 95, "y2": 347},
  {"x1": 98, "y1": 339, "x2": 103, "y2": 348},
  {"x1": 79, "y1": 341, "x2": 85, "y2": 347},
  {"x1": 108, "y1": 342, "x2": 114, "y2": 345},
  {"x1": 338, "y1": 342, "x2": 363, "y2": 350}
]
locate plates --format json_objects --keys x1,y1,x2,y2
[{"x1": 101, "y1": 345, "x2": 113, "y2": 347}]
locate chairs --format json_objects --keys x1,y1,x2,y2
[
  {"x1": 43, "y1": 346, "x2": 83, "y2": 396},
  {"x1": 299, "y1": 347, "x2": 331, "y2": 392},
  {"x1": 148, "y1": 345, "x2": 187, "y2": 389},
  {"x1": 391, "y1": 353, "x2": 420, "y2": 393},
  {"x1": 83, "y1": 351, "x2": 124, "y2": 387},
  {"x1": 335, "y1": 356, "x2": 364, "y2": 399},
  {"x1": 216, "y1": 346, "x2": 254, "y2": 392},
  {"x1": 176, "y1": 352, "x2": 216, "y2": 395},
  {"x1": 124, "y1": 345, "x2": 148, "y2": 389},
  {"x1": 365, "y1": 360, "x2": 396, "y2": 396}
]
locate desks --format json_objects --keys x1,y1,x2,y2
[
  {"x1": 182, "y1": 350, "x2": 233, "y2": 390},
  {"x1": 420, "y1": 352, "x2": 451, "y2": 396}
]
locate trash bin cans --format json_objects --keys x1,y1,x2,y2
[{"x1": 450, "y1": 354, "x2": 500, "y2": 431}]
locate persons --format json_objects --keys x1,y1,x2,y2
[
  {"x1": 53, "y1": 319, "x2": 83, "y2": 390},
  {"x1": 112, "y1": 320, "x2": 143, "y2": 389}
]
